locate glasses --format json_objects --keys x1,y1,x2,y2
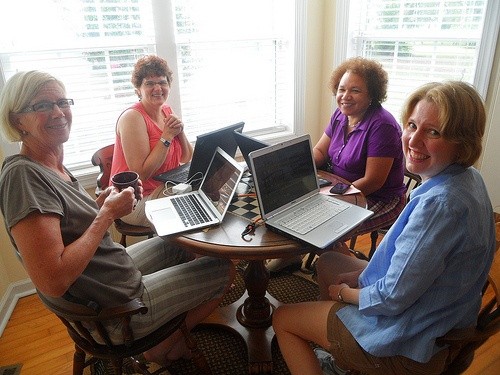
[
  {"x1": 141, "y1": 80, "x2": 169, "y2": 88},
  {"x1": 19, "y1": 99, "x2": 74, "y2": 113}
]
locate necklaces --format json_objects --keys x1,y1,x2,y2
[{"x1": 348, "y1": 120, "x2": 360, "y2": 128}]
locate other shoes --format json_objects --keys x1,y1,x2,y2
[
  {"x1": 313, "y1": 348, "x2": 350, "y2": 375},
  {"x1": 264, "y1": 255, "x2": 303, "y2": 274}
]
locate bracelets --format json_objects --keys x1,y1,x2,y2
[{"x1": 338, "y1": 286, "x2": 347, "y2": 301}]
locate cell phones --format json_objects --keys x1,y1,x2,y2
[{"x1": 329, "y1": 182, "x2": 351, "y2": 194}]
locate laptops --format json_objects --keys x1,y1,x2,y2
[
  {"x1": 152, "y1": 122, "x2": 245, "y2": 186},
  {"x1": 144, "y1": 146, "x2": 245, "y2": 237},
  {"x1": 249, "y1": 133, "x2": 374, "y2": 249},
  {"x1": 233, "y1": 130, "x2": 331, "y2": 187}
]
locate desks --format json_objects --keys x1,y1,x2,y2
[{"x1": 146, "y1": 165, "x2": 370, "y2": 375}]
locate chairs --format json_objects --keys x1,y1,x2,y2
[
  {"x1": 431, "y1": 272, "x2": 500, "y2": 375},
  {"x1": 34, "y1": 285, "x2": 212, "y2": 375},
  {"x1": 91, "y1": 144, "x2": 155, "y2": 248},
  {"x1": 305, "y1": 167, "x2": 422, "y2": 272}
]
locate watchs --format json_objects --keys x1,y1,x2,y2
[{"x1": 160, "y1": 137, "x2": 171, "y2": 147}]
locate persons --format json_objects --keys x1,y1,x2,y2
[
  {"x1": 109, "y1": 54, "x2": 194, "y2": 229},
  {"x1": 263, "y1": 57, "x2": 406, "y2": 277},
  {"x1": 0, "y1": 70, "x2": 236, "y2": 375},
  {"x1": 272, "y1": 80, "x2": 496, "y2": 375}
]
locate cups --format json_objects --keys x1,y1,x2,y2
[{"x1": 111, "y1": 171, "x2": 139, "y2": 199}]
